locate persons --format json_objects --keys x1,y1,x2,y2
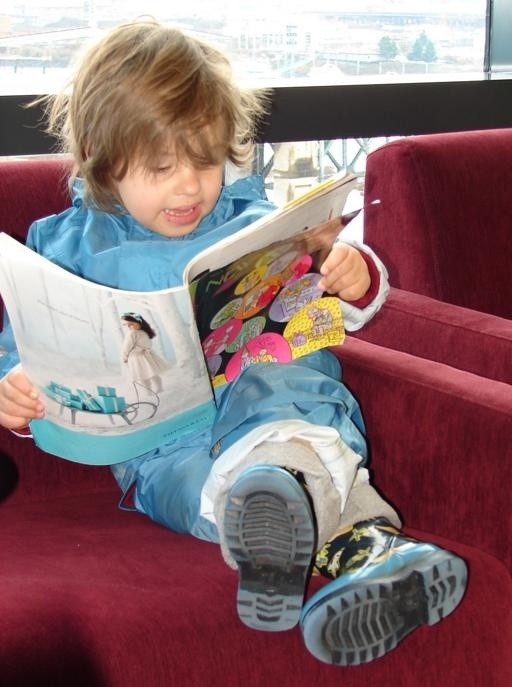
[{"x1": 0, "y1": 11, "x2": 470, "y2": 668}]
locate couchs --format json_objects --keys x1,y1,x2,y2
[
  {"x1": 0, "y1": 152, "x2": 512, "y2": 685},
  {"x1": 344, "y1": 127, "x2": 512, "y2": 386}
]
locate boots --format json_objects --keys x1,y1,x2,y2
[{"x1": 298, "y1": 514, "x2": 469, "y2": 667}]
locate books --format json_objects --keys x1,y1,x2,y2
[{"x1": 0, "y1": 167, "x2": 367, "y2": 467}]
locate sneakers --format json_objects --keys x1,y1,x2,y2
[{"x1": 221, "y1": 464, "x2": 319, "y2": 633}]
